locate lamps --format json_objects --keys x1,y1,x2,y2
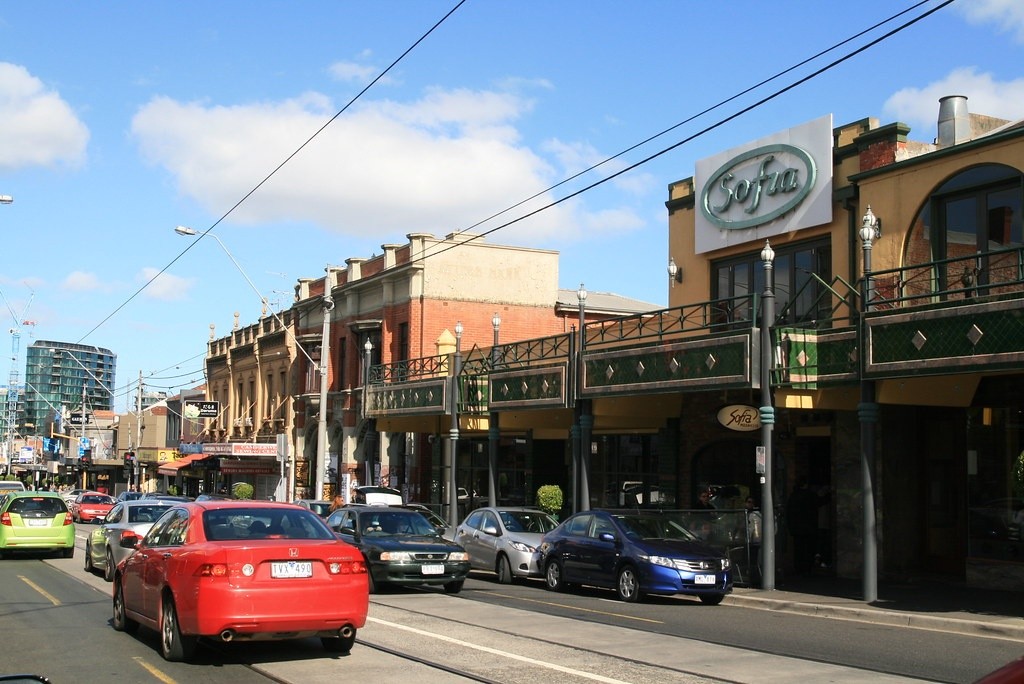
[
  {"x1": 871, "y1": 212, "x2": 882, "y2": 239},
  {"x1": 667, "y1": 256, "x2": 682, "y2": 288}
]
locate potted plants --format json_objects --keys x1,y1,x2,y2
[{"x1": 535, "y1": 484, "x2": 563, "y2": 530}]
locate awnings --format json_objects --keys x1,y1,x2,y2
[{"x1": 158, "y1": 454, "x2": 213, "y2": 476}]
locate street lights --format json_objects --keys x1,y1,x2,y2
[
  {"x1": 11, "y1": 381, "x2": 87, "y2": 494},
  {"x1": 175, "y1": 227, "x2": 334, "y2": 514},
  {"x1": 49, "y1": 348, "x2": 142, "y2": 447}
]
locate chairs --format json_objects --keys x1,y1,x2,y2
[
  {"x1": 287, "y1": 527, "x2": 306, "y2": 538},
  {"x1": 132, "y1": 514, "x2": 151, "y2": 522},
  {"x1": 214, "y1": 527, "x2": 237, "y2": 539}
]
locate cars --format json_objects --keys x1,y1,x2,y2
[
  {"x1": 62, "y1": 489, "x2": 236, "y2": 581},
  {"x1": 0, "y1": 481, "x2": 75, "y2": 558},
  {"x1": 458, "y1": 487, "x2": 478, "y2": 501},
  {"x1": 113, "y1": 499, "x2": 370, "y2": 662},
  {"x1": 291, "y1": 497, "x2": 472, "y2": 594},
  {"x1": 454, "y1": 507, "x2": 559, "y2": 584},
  {"x1": 540, "y1": 510, "x2": 732, "y2": 606}
]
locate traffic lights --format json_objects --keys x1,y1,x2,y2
[
  {"x1": 45, "y1": 421, "x2": 54, "y2": 439},
  {"x1": 82, "y1": 457, "x2": 89, "y2": 470},
  {"x1": 123, "y1": 451, "x2": 131, "y2": 469}
]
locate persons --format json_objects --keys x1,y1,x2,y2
[
  {"x1": 784, "y1": 476, "x2": 831, "y2": 577},
  {"x1": 327, "y1": 495, "x2": 344, "y2": 517},
  {"x1": 363, "y1": 520, "x2": 381, "y2": 532},
  {"x1": 676, "y1": 490, "x2": 762, "y2": 589}
]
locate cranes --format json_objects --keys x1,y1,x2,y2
[{"x1": 0, "y1": 281, "x2": 36, "y2": 441}]
